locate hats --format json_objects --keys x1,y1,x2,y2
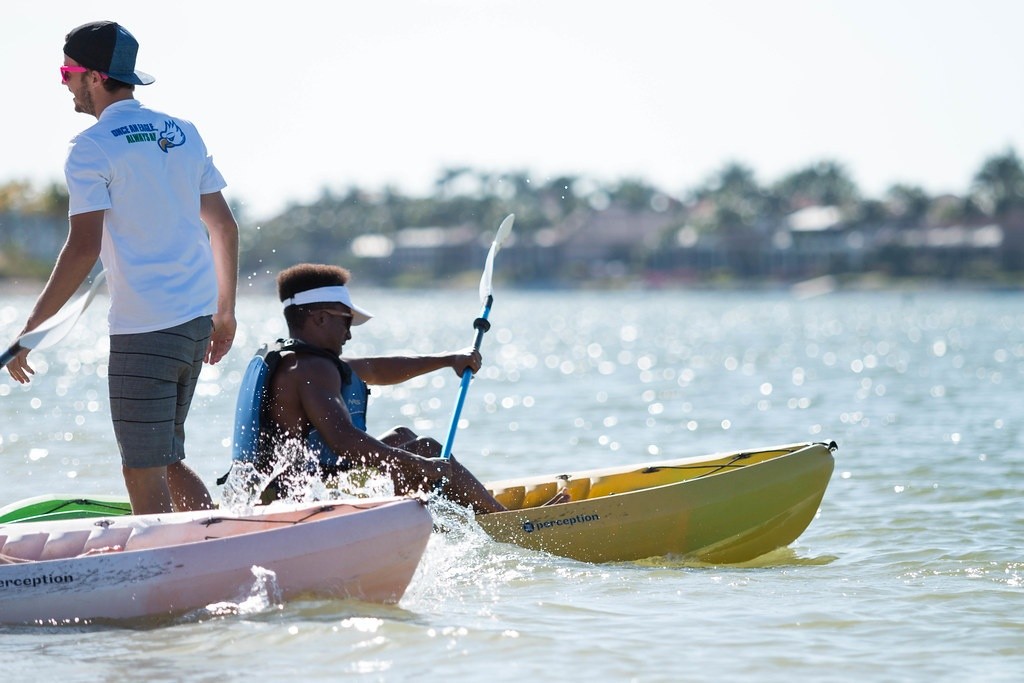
[
  {"x1": 62, "y1": 21, "x2": 155, "y2": 85},
  {"x1": 282, "y1": 286, "x2": 372, "y2": 327}
]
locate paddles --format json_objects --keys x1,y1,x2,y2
[
  {"x1": 0, "y1": 267, "x2": 109, "y2": 370},
  {"x1": 440, "y1": 213, "x2": 514, "y2": 458}
]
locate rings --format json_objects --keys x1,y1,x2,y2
[{"x1": 474, "y1": 359, "x2": 476, "y2": 363}]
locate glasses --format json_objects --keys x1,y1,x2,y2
[
  {"x1": 59, "y1": 66, "x2": 109, "y2": 82},
  {"x1": 308, "y1": 310, "x2": 353, "y2": 329}
]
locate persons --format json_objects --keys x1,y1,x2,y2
[
  {"x1": 217, "y1": 263, "x2": 570, "y2": 513},
  {"x1": 6, "y1": 20, "x2": 240, "y2": 515}
]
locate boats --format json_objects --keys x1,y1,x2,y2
[
  {"x1": 1, "y1": 496, "x2": 433, "y2": 623},
  {"x1": 1, "y1": 438, "x2": 837, "y2": 568}
]
